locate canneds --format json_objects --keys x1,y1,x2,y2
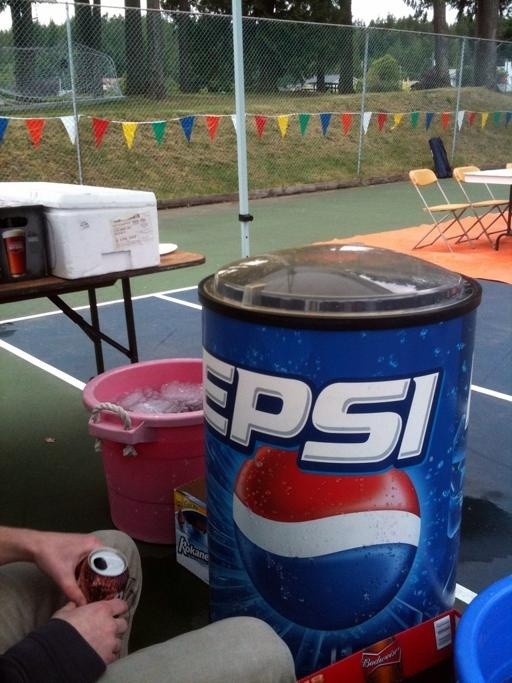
[{"x1": 76, "y1": 546, "x2": 130, "y2": 602}]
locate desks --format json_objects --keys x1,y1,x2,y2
[{"x1": 0, "y1": 250, "x2": 206, "y2": 375}]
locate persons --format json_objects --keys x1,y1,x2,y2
[{"x1": 0, "y1": 525, "x2": 298, "y2": 683}]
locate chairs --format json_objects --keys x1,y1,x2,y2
[{"x1": 409, "y1": 163, "x2": 512, "y2": 255}]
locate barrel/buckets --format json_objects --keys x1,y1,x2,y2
[
  {"x1": 198, "y1": 244, "x2": 483, "y2": 683},
  {"x1": 454, "y1": 573, "x2": 512, "y2": 682},
  {"x1": 84, "y1": 358, "x2": 204, "y2": 543}
]
